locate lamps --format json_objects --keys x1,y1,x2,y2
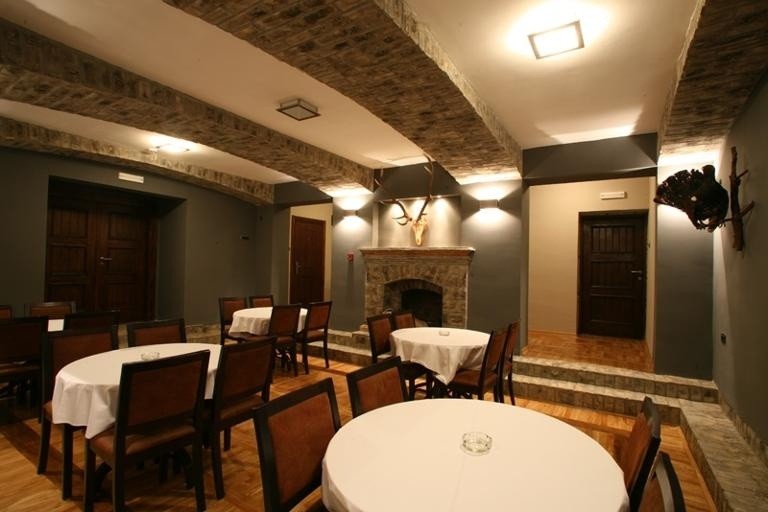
[
  {"x1": 476, "y1": 198, "x2": 500, "y2": 210},
  {"x1": 275, "y1": 97, "x2": 321, "y2": 122},
  {"x1": 342, "y1": 207, "x2": 358, "y2": 219}
]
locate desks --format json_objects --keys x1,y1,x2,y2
[
  {"x1": 318, "y1": 395, "x2": 632, "y2": 511},
  {"x1": 388, "y1": 324, "x2": 491, "y2": 402},
  {"x1": 52, "y1": 343, "x2": 222, "y2": 498}
]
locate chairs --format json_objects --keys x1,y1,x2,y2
[
  {"x1": 636, "y1": 451, "x2": 689, "y2": 511},
  {"x1": 449, "y1": 326, "x2": 508, "y2": 405},
  {"x1": 201, "y1": 337, "x2": 278, "y2": 500},
  {"x1": 219, "y1": 295, "x2": 333, "y2": 377},
  {"x1": 347, "y1": 354, "x2": 408, "y2": 417},
  {"x1": 619, "y1": 395, "x2": 661, "y2": 510},
  {"x1": 127, "y1": 318, "x2": 186, "y2": 347},
  {"x1": 83, "y1": 349, "x2": 206, "y2": 512},
  {"x1": 252, "y1": 378, "x2": 340, "y2": 512},
  {"x1": 391, "y1": 310, "x2": 415, "y2": 331},
  {"x1": 470, "y1": 319, "x2": 521, "y2": 406},
  {"x1": 365, "y1": 310, "x2": 433, "y2": 400},
  {"x1": 0, "y1": 288, "x2": 120, "y2": 422},
  {"x1": 37, "y1": 325, "x2": 117, "y2": 500}
]
